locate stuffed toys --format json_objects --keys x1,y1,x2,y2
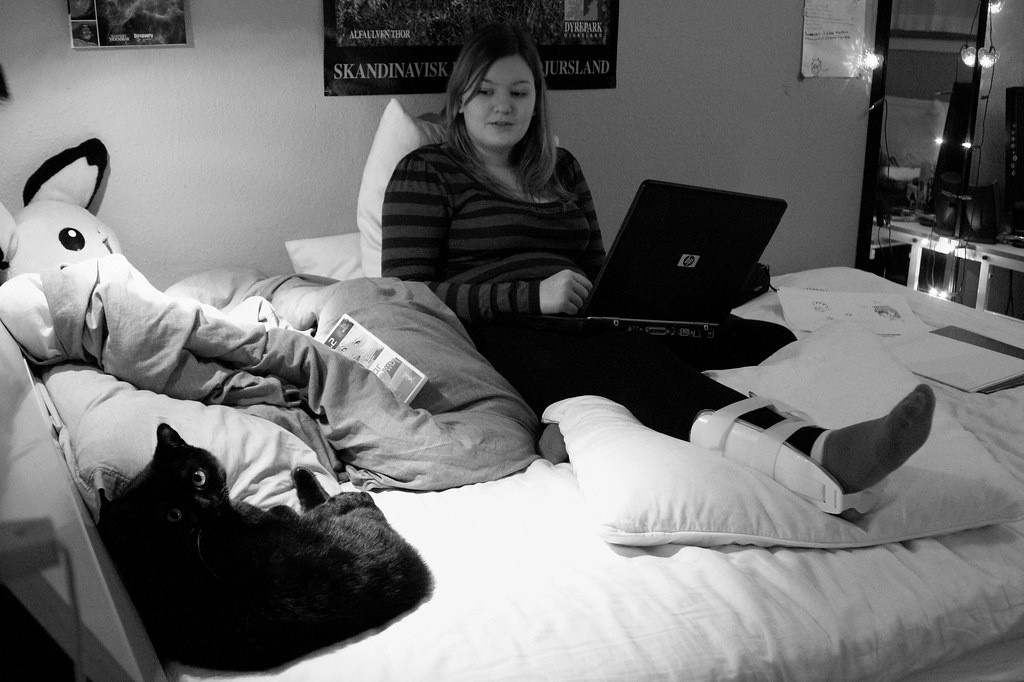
[{"x1": 0, "y1": 137, "x2": 122, "y2": 281}]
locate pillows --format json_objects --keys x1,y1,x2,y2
[
  {"x1": 285, "y1": 234, "x2": 372, "y2": 287},
  {"x1": 41, "y1": 362, "x2": 340, "y2": 518},
  {"x1": 356, "y1": 98, "x2": 560, "y2": 278},
  {"x1": 541, "y1": 319, "x2": 1024, "y2": 547}
]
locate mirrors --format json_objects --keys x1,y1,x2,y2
[{"x1": 853, "y1": 0, "x2": 990, "y2": 298}]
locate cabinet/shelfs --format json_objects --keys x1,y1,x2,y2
[{"x1": 871, "y1": 216, "x2": 1024, "y2": 322}]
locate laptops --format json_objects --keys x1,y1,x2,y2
[{"x1": 541, "y1": 179, "x2": 789, "y2": 339}]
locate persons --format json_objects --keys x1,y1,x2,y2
[
  {"x1": 340, "y1": 326, "x2": 378, "y2": 370},
  {"x1": 381, "y1": 23, "x2": 936, "y2": 522}
]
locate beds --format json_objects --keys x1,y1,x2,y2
[{"x1": 0, "y1": 139, "x2": 1024, "y2": 682}]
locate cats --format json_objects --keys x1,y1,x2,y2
[{"x1": 97, "y1": 423, "x2": 434, "y2": 673}]
